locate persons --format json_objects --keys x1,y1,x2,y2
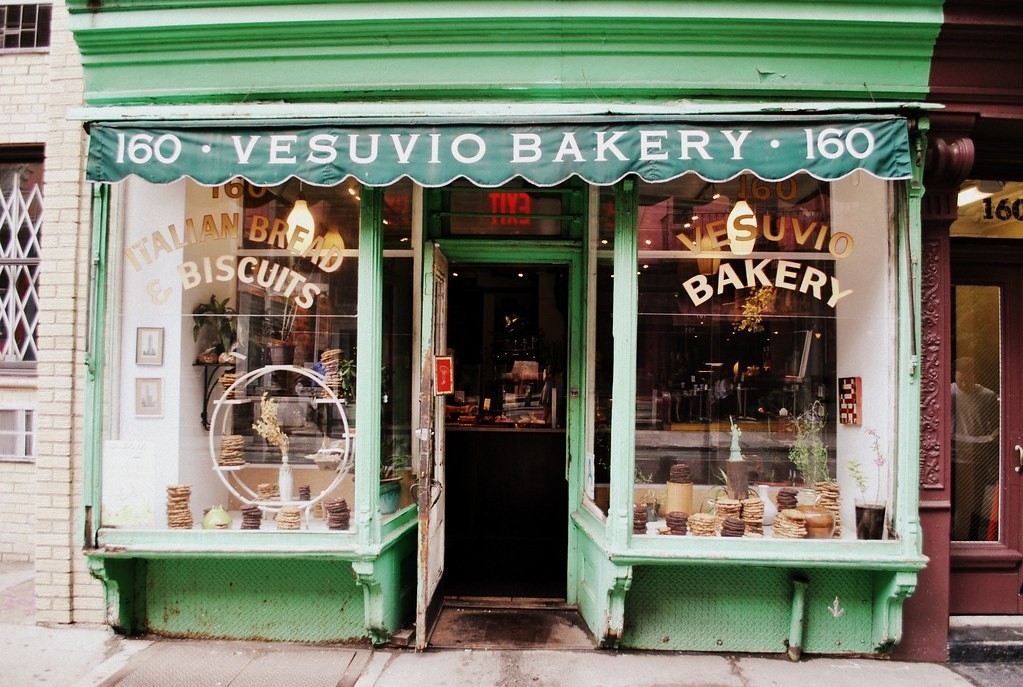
[{"x1": 949, "y1": 357, "x2": 999, "y2": 540}]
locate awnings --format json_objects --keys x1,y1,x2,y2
[{"x1": 82, "y1": 116, "x2": 917, "y2": 187}]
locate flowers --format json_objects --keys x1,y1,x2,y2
[{"x1": 253, "y1": 393, "x2": 292, "y2": 454}]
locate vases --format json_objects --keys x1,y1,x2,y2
[{"x1": 278, "y1": 456, "x2": 295, "y2": 504}]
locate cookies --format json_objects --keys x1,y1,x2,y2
[
  {"x1": 219, "y1": 435, "x2": 246, "y2": 466},
  {"x1": 165, "y1": 483, "x2": 193, "y2": 530},
  {"x1": 240, "y1": 482, "x2": 350, "y2": 529},
  {"x1": 632, "y1": 464, "x2": 842, "y2": 538},
  {"x1": 218, "y1": 371, "x2": 249, "y2": 398},
  {"x1": 320, "y1": 348, "x2": 343, "y2": 398}
]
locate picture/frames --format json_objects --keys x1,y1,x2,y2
[
  {"x1": 136, "y1": 327, "x2": 164, "y2": 366},
  {"x1": 135, "y1": 377, "x2": 164, "y2": 417}
]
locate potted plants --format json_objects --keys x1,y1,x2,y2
[
  {"x1": 379, "y1": 444, "x2": 414, "y2": 515},
  {"x1": 340, "y1": 361, "x2": 386, "y2": 429},
  {"x1": 191, "y1": 294, "x2": 243, "y2": 355}
]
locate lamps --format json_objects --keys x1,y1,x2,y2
[
  {"x1": 285, "y1": 179, "x2": 314, "y2": 253},
  {"x1": 318, "y1": 229, "x2": 344, "y2": 271},
  {"x1": 699, "y1": 230, "x2": 722, "y2": 276},
  {"x1": 729, "y1": 175, "x2": 757, "y2": 256}
]
